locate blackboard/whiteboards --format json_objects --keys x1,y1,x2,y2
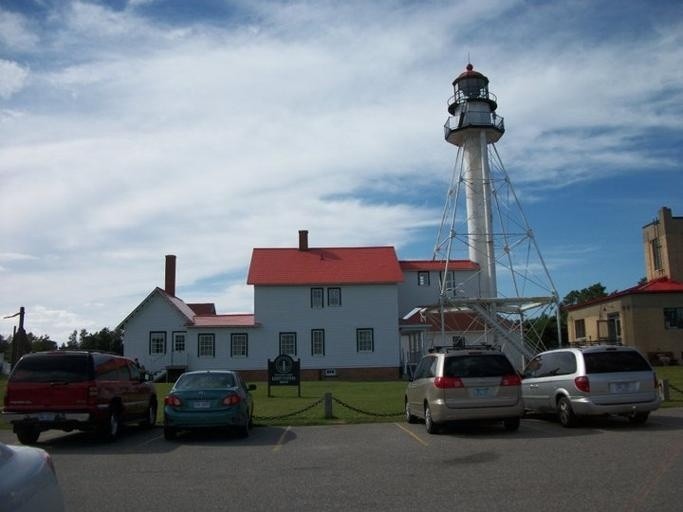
[{"x1": 647, "y1": 351, "x2": 681, "y2": 367}]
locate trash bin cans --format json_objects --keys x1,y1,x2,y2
[{"x1": 165, "y1": 366, "x2": 186, "y2": 383}]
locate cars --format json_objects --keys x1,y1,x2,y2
[
  {"x1": 164, "y1": 370, "x2": 256, "y2": 441},
  {"x1": 0, "y1": 443, "x2": 63, "y2": 512}
]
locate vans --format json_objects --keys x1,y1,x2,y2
[
  {"x1": 405, "y1": 345, "x2": 660, "y2": 433},
  {"x1": 1, "y1": 350, "x2": 158, "y2": 444}
]
